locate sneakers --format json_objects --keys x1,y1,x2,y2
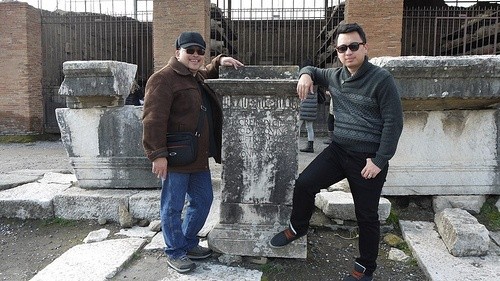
[
  {"x1": 269, "y1": 228, "x2": 308, "y2": 248},
  {"x1": 345, "y1": 258, "x2": 374, "y2": 281},
  {"x1": 165, "y1": 244, "x2": 213, "y2": 273}
]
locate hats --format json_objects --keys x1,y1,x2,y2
[{"x1": 175, "y1": 32, "x2": 206, "y2": 51}]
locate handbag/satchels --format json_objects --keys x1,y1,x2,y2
[{"x1": 166, "y1": 132, "x2": 199, "y2": 166}]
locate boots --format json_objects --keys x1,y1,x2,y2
[
  {"x1": 323, "y1": 130, "x2": 334, "y2": 144},
  {"x1": 300, "y1": 141, "x2": 314, "y2": 153}
]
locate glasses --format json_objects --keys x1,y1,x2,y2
[
  {"x1": 185, "y1": 48, "x2": 206, "y2": 55},
  {"x1": 335, "y1": 42, "x2": 364, "y2": 54}
]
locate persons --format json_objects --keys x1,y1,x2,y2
[
  {"x1": 300, "y1": 60, "x2": 319, "y2": 152},
  {"x1": 323, "y1": 91, "x2": 334, "y2": 144},
  {"x1": 269, "y1": 24, "x2": 403, "y2": 281},
  {"x1": 142, "y1": 32, "x2": 244, "y2": 273}
]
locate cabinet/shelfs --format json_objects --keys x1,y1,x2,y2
[
  {"x1": 441, "y1": 5, "x2": 500, "y2": 55},
  {"x1": 210, "y1": 4, "x2": 237, "y2": 60},
  {"x1": 314, "y1": 2, "x2": 345, "y2": 67}
]
